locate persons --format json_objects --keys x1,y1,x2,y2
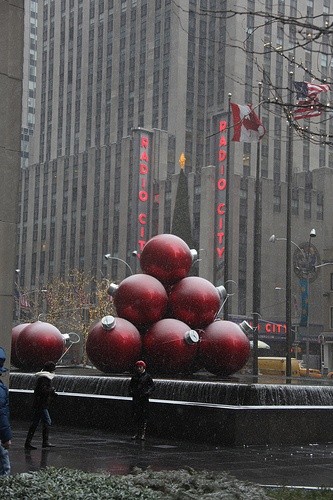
[
  {"x1": 129, "y1": 360, "x2": 156, "y2": 440},
  {"x1": 25, "y1": 361, "x2": 58, "y2": 449},
  {"x1": 0, "y1": 347, "x2": 13, "y2": 475}
]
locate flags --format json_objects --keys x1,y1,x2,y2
[
  {"x1": 230, "y1": 102, "x2": 266, "y2": 142},
  {"x1": 292, "y1": 81, "x2": 330, "y2": 120}
]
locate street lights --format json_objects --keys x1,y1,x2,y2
[
  {"x1": 268, "y1": 228, "x2": 333, "y2": 377},
  {"x1": 15, "y1": 269, "x2": 48, "y2": 325},
  {"x1": 275, "y1": 287, "x2": 299, "y2": 359}
]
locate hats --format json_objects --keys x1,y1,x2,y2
[
  {"x1": 137, "y1": 361, "x2": 146, "y2": 368},
  {"x1": 43, "y1": 361, "x2": 56, "y2": 372}
]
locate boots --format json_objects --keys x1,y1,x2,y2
[{"x1": 131, "y1": 422, "x2": 146, "y2": 440}]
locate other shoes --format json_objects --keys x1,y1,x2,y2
[
  {"x1": 25, "y1": 445, "x2": 37, "y2": 450},
  {"x1": 42, "y1": 444, "x2": 56, "y2": 447}
]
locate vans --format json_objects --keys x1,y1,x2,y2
[{"x1": 258, "y1": 356, "x2": 301, "y2": 384}]
